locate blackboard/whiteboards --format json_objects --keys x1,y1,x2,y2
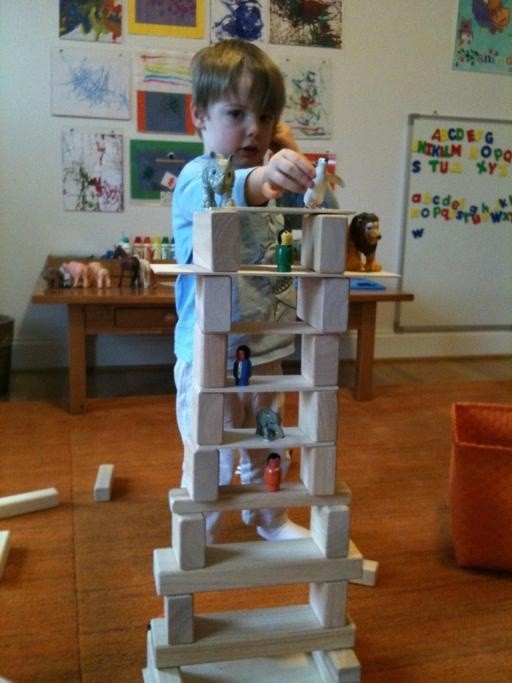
[{"x1": 394, "y1": 113, "x2": 512, "y2": 333}]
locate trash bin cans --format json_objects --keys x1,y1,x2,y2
[{"x1": 451, "y1": 402, "x2": 512, "y2": 572}]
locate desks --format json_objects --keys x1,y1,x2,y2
[{"x1": 32, "y1": 254, "x2": 413, "y2": 412}]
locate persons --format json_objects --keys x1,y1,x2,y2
[{"x1": 172, "y1": 40, "x2": 339, "y2": 546}]
